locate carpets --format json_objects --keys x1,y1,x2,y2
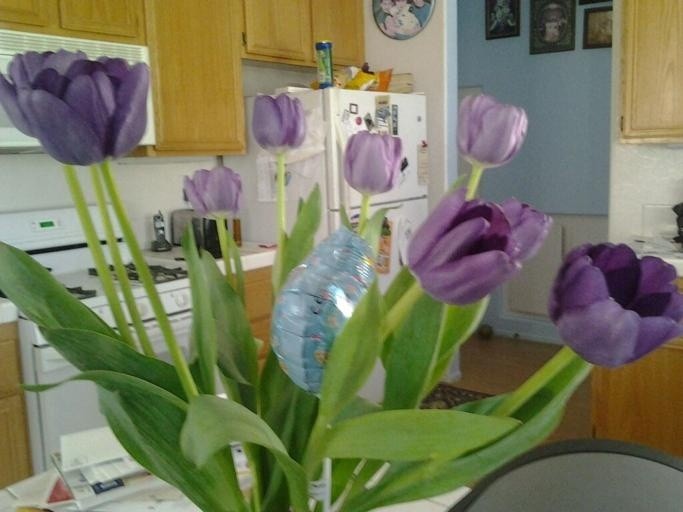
[{"x1": 421, "y1": 381, "x2": 496, "y2": 409}]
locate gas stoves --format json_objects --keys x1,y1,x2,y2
[{"x1": 1, "y1": 260, "x2": 198, "y2": 346}]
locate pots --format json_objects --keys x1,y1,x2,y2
[{"x1": 670, "y1": 202, "x2": 682, "y2": 246}]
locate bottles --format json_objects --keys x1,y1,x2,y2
[{"x1": 230, "y1": 211, "x2": 242, "y2": 249}]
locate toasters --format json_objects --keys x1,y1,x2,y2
[{"x1": 170, "y1": 208, "x2": 195, "y2": 248}]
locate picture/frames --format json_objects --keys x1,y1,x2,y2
[
  {"x1": 372, "y1": 0, "x2": 435, "y2": 40},
  {"x1": 583, "y1": 6, "x2": 612, "y2": 49},
  {"x1": 485, "y1": 0, "x2": 520, "y2": 40},
  {"x1": 529, "y1": 0, "x2": 575, "y2": 54}
]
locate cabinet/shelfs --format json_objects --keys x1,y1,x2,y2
[
  {"x1": 225, "y1": 265, "x2": 274, "y2": 359},
  {"x1": 619, "y1": 0, "x2": 683, "y2": 144},
  {"x1": 240, "y1": 1, "x2": 364, "y2": 72},
  {"x1": 0, "y1": 321, "x2": 33, "y2": 489},
  {"x1": 127, "y1": 0, "x2": 246, "y2": 157},
  {"x1": 0, "y1": 0, "x2": 146, "y2": 45}
]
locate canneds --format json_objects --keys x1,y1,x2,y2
[{"x1": 313, "y1": 40, "x2": 333, "y2": 89}]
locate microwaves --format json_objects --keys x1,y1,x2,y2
[{"x1": 0, "y1": 30, "x2": 156, "y2": 153}]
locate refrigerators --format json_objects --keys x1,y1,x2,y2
[{"x1": 237, "y1": 87, "x2": 430, "y2": 409}]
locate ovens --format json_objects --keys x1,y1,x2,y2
[{"x1": 21, "y1": 309, "x2": 196, "y2": 474}]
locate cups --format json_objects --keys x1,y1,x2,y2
[{"x1": 192, "y1": 217, "x2": 228, "y2": 259}]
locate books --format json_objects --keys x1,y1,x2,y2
[{"x1": 43, "y1": 424, "x2": 168, "y2": 512}]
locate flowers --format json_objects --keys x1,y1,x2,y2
[{"x1": 0, "y1": 47, "x2": 683, "y2": 512}]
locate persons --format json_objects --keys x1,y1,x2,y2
[{"x1": 375, "y1": 0, "x2": 431, "y2": 36}]
